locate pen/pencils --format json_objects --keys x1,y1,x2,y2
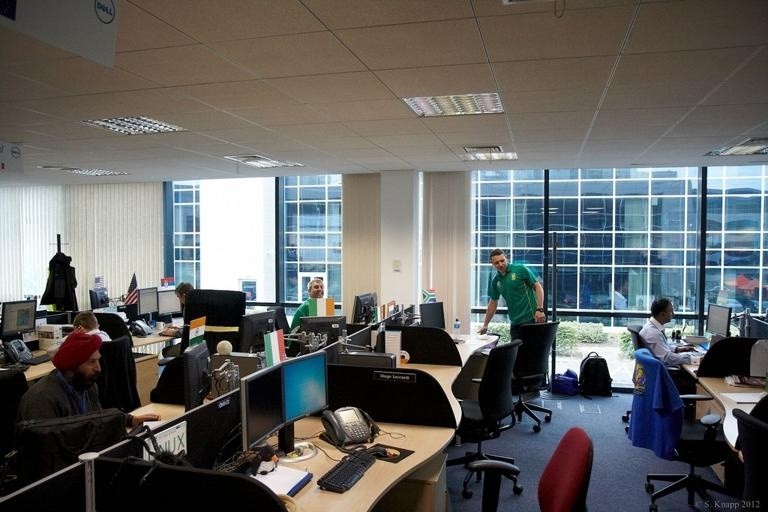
[{"x1": 142, "y1": 318, "x2": 146, "y2": 324}]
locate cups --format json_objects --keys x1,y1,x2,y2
[
  {"x1": 689, "y1": 356, "x2": 700, "y2": 366},
  {"x1": 155, "y1": 322, "x2": 165, "y2": 330}
]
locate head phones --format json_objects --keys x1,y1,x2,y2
[{"x1": 234, "y1": 443, "x2": 280, "y2": 475}]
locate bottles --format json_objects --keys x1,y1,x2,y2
[
  {"x1": 671, "y1": 329, "x2": 681, "y2": 342},
  {"x1": 452, "y1": 318, "x2": 461, "y2": 339}
]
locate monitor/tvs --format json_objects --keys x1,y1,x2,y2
[
  {"x1": 706, "y1": 304, "x2": 732, "y2": 339},
  {"x1": 351, "y1": 293, "x2": 377, "y2": 324},
  {"x1": 419, "y1": 301, "x2": 445, "y2": 330},
  {"x1": 299, "y1": 315, "x2": 346, "y2": 350},
  {"x1": 281, "y1": 352, "x2": 329, "y2": 430},
  {"x1": 183, "y1": 340, "x2": 213, "y2": 412},
  {"x1": 1, "y1": 299, "x2": 36, "y2": 340},
  {"x1": 137, "y1": 287, "x2": 159, "y2": 323},
  {"x1": 238, "y1": 367, "x2": 285, "y2": 453},
  {"x1": 89, "y1": 288, "x2": 109, "y2": 309},
  {"x1": 157, "y1": 290, "x2": 182, "y2": 322}
]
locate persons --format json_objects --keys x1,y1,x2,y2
[
  {"x1": 47, "y1": 311, "x2": 112, "y2": 359},
  {"x1": 287, "y1": 278, "x2": 325, "y2": 348},
  {"x1": 610, "y1": 277, "x2": 628, "y2": 311},
  {"x1": 9, "y1": 324, "x2": 161, "y2": 474},
  {"x1": 159, "y1": 282, "x2": 195, "y2": 358},
  {"x1": 477, "y1": 249, "x2": 546, "y2": 401},
  {"x1": 637, "y1": 298, "x2": 704, "y2": 367}
]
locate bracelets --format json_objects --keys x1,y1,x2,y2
[{"x1": 535, "y1": 308, "x2": 545, "y2": 312}]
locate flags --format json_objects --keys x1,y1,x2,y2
[
  {"x1": 188, "y1": 317, "x2": 206, "y2": 348},
  {"x1": 374, "y1": 305, "x2": 383, "y2": 323},
  {"x1": 95, "y1": 276, "x2": 105, "y2": 289},
  {"x1": 422, "y1": 288, "x2": 436, "y2": 303},
  {"x1": 124, "y1": 273, "x2": 137, "y2": 305},
  {"x1": 263, "y1": 329, "x2": 286, "y2": 367},
  {"x1": 308, "y1": 298, "x2": 335, "y2": 317}
]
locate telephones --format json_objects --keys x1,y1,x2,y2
[
  {"x1": 129, "y1": 320, "x2": 154, "y2": 336},
  {"x1": 2, "y1": 339, "x2": 33, "y2": 364},
  {"x1": 320, "y1": 406, "x2": 380, "y2": 447}
]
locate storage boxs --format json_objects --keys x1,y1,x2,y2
[{"x1": 550, "y1": 369, "x2": 578, "y2": 395}]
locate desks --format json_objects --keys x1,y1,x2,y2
[
  {"x1": 0, "y1": 312, "x2": 498, "y2": 512},
  {"x1": 675, "y1": 337, "x2": 768, "y2": 460}
]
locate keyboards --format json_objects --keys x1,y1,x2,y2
[
  {"x1": 452, "y1": 339, "x2": 465, "y2": 345},
  {"x1": 317, "y1": 451, "x2": 376, "y2": 493}
]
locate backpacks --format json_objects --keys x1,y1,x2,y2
[{"x1": 578, "y1": 352, "x2": 612, "y2": 400}]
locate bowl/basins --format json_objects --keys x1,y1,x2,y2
[{"x1": 687, "y1": 337, "x2": 707, "y2": 344}]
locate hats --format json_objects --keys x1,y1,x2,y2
[{"x1": 52, "y1": 330, "x2": 102, "y2": 370}]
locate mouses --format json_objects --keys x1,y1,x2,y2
[{"x1": 367, "y1": 446, "x2": 388, "y2": 458}]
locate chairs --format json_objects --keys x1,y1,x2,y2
[
  {"x1": 538, "y1": 426, "x2": 594, "y2": 512},
  {"x1": 622, "y1": 326, "x2": 684, "y2": 423},
  {"x1": 733, "y1": 408, "x2": 768, "y2": 512},
  {"x1": 630, "y1": 348, "x2": 742, "y2": 512},
  {"x1": 472, "y1": 320, "x2": 560, "y2": 432},
  {"x1": 447, "y1": 340, "x2": 524, "y2": 499}
]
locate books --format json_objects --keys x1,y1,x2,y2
[
  {"x1": 719, "y1": 392, "x2": 767, "y2": 403},
  {"x1": 237, "y1": 461, "x2": 314, "y2": 498},
  {"x1": 725, "y1": 375, "x2": 766, "y2": 388}
]
